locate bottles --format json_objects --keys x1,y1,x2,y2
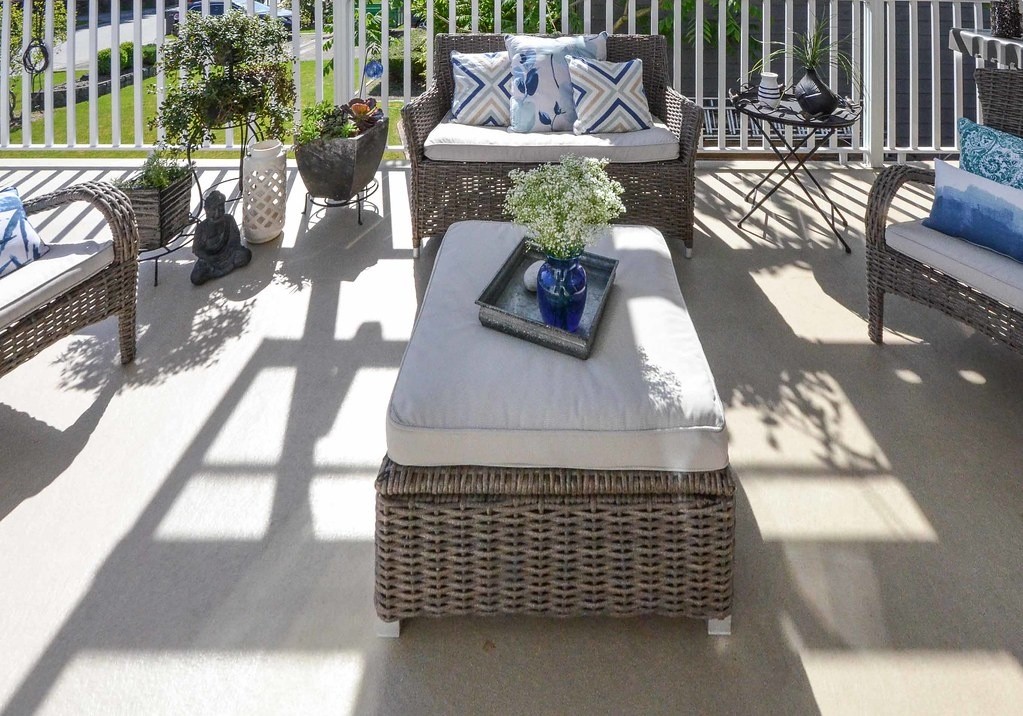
[{"x1": 758, "y1": 72, "x2": 780, "y2": 108}]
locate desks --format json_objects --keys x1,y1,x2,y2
[{"x1": 949, "y1": 29, "x2": 1023, "y2": 70}]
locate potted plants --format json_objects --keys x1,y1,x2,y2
[
  {"x1": 112, "y1": 9, "x2": 389, "y2": 249},
  {"x1": 736, "y1": 9, "x2": 864, "y2": 114}
]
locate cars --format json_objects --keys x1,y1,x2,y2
[{"x1": 165, "y1": 0, "x2": 301, "y2": 34}]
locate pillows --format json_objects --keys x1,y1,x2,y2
[
  {"x1": 565, "y1": 55, "x2": 653, "y2": 136},
  {"x1": 0, "y1": 187, "x2": 49, "y2": 280},
  {"x1": 957, "y1": 118, "x2": 1023, "y2": 190},
  {"x1": 922, "y1": 157, "x2": 1023, "y2": 264},
  {"x1": 504, "y1": 31, "x2": 609, "y2": 132},
  {"x1": 450, "y1": 50, "x2": 511, "y2": 127}
]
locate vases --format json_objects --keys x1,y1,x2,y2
[
  {"x1": 758, "y1": 72, "x2": 780, "y2": 107},
  {"x1": 990, "y1": 0, "x2": 1022, "y2": 39},
  {"x1": 537, "y1": 252, "x2": 587, "y2": 333}
]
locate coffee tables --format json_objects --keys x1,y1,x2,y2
[
  {"x1": 371, "y1": 220, "x2": 736, "y2": 637},
  {"x1": 729, "y1": 88, "x2": 863, "y2": 254}
]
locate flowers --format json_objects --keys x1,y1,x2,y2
[{"x1": 500, "y1": 152, "x2": 625, "y2": 327}]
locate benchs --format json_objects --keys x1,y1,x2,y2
[
  {"x1": 864, "y1": 68, "x2": 1023, "y2": 356},
  {"x1": 401, "y1": 34, "x2": 705, "y2": 258},
  {"x1": 0, "y1": 180, "x2": 138, "y2": 379}
]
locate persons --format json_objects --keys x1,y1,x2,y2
[{"x1": 189, "y1": 191, "x2": 252, "y2": 286}]
